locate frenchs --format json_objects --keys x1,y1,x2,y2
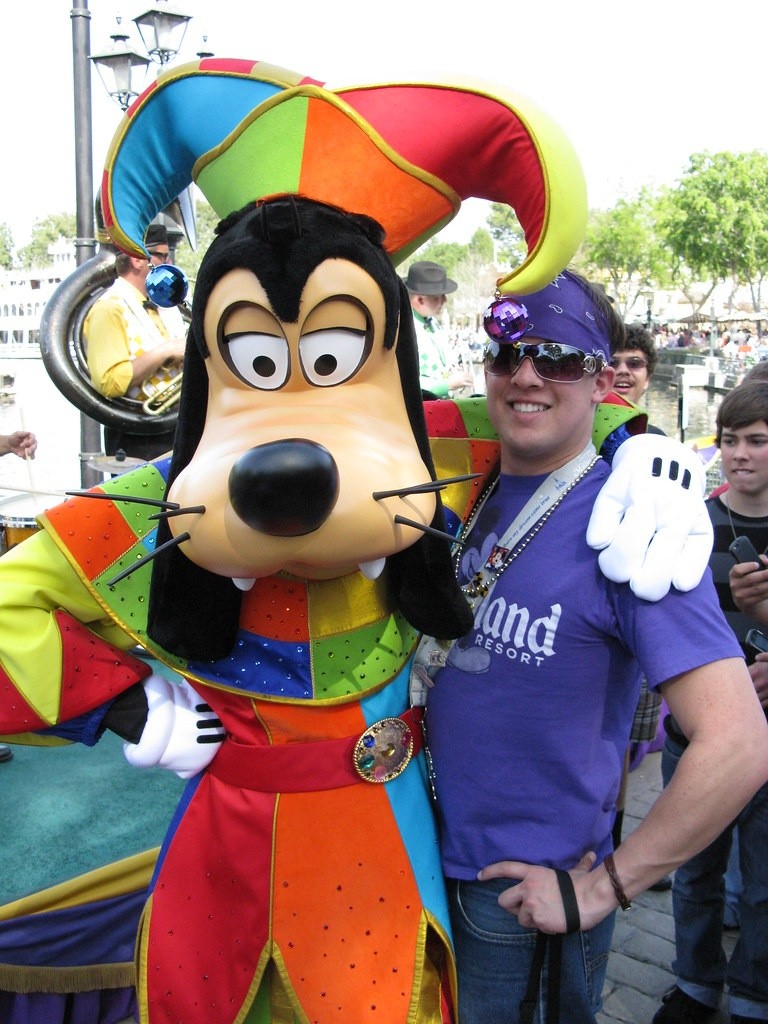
[{"x1": 38, "y1": 184, "x2": 199, "y2": 436}]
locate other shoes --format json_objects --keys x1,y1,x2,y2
[
  {"x1": 651, "y1": 983, "x2": 768, "y2": 1024},
  {"x1": 650, "y1": 873, "x2": 672, "y2": 891}
]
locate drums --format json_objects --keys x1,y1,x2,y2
[{"x1": 0, "y1": 489, "x2": 80, "y2": 550}]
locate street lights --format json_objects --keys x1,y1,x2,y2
[
  {"x1": 88, "y1": 0, "x2": 223, "y2": 270},
  {"x1": 646, "y1": 299, "x2": 653, "y2": 329}
]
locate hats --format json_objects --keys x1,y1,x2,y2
[
  {"x1": 401, "y1": 261, "x2": 458, "y2": 295},
  {"x1": 114, "y1": 224, "x2": 169, "y2": 256}
]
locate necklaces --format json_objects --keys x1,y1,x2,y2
[
  {"x1": 408, "y1": 439, "x2": 601, "y2": 803},
  {"x1": 725, "y1": 492, "x2": 767, "y2": 557},
  {"x1": 454, "y1": 452, "x2": 603, "y2": 595}
]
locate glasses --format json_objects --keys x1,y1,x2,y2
[
  {"x1": 482, "y1": 339, "x2": 609, "y2": 384},
  {"x1": 610, "y1": 357, "x2": 649, "y2": 368},
  {"x1": 149, "y1": 252, "x2": 170, "y2": 263}
]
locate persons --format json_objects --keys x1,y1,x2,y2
[
  {"x1": 422, "y1": 265, "x2": 768, "y2": 1024},
  {"x1": 609, "y1": 323, "x2": 669, "y2": 437},
  {"x1": 648, "y1": 384, "x2": 768, "y2": 1024},
  {"x1": 0, "y1": 430, "x2": 38, "y2": 461},
  {"x1": 399, "y1": 261, "x2": 474, "y2": 401},
  {"x1": 82, "y1": 224, "x2": 187, "y2": 478},
  {"x1": 728, "y1": 552, "x2": 768, "y2": 626},
  {"x1": 645, "y1": 321, "x2": 768, "y2": 360}
]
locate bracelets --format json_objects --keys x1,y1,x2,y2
[{"x1": 603, "y1": 852, "x2": 633, "y2": 912}]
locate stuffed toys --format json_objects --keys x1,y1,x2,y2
[{"x1": 0, "y1": 56, "x2": 716, "y2": 1024}]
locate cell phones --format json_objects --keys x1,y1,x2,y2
[
  {"x1": 729, "y1": 536, "x2": 766, "y2": 573},
  {"x1": 744, "y1": 629, "x2": 768, "y2": 654}
]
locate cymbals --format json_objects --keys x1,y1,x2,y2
[{"x1": 85, "y1": 455, "x2": 149, "y2": 475}]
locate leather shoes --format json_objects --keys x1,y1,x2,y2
[{"x1": 0, "y1": 745, "x2": 13, "y2": 763}]
